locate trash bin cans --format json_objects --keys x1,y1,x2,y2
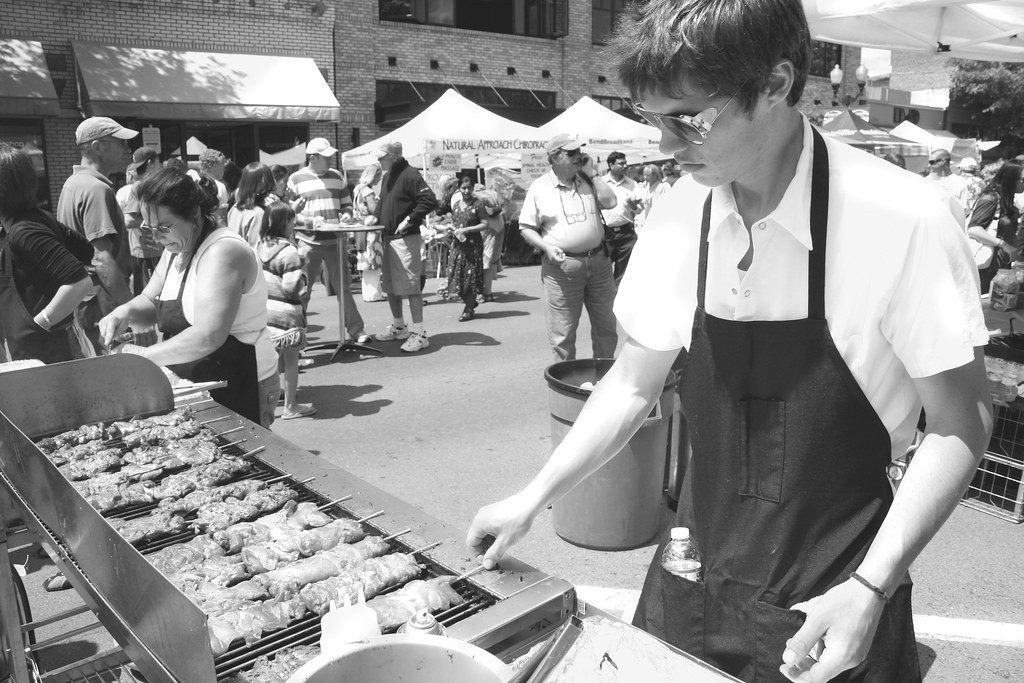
[{"x1": 542, "y1": 357, "x2": 681, "y2": 553}]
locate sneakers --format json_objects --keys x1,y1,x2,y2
[
  {"x1": 399, "y1": 330, "x2": 430, "y2": 352},
  {"x1": 376, "y1": 323, "x2": 409, "y2": 340},
  {"x1": 349, "y1": 329, "x2": 372, "y2": 343}
]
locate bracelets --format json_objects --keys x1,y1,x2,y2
[
  {"x1": 42, "y1": 309, "x2": 53, "y2": 326},
  {"x1": 848, "y1": 572, "x2": 892, "y2": 604}
]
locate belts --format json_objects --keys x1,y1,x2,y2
[
  {"x1": 609, "y1": 222, "x2": 632, "y2": 231},
  {"x1": 565, "y1": 245, "x2": 604, "y2": 257}
]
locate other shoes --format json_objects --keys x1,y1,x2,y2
[
  {"x1": 459, "y1": 301, "x2": 479, "y2": 322},
  {"x1": 476, "y1": 292, "x2": 494, "y2": 303}
]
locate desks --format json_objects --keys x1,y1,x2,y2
[{"x1": 294, "y1": 225, "x2": 386, "y2": 363}]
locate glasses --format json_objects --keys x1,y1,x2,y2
[
  {"x1": 101, "y1": 138, "x2": 128, "y2": 146},
  {"x1": 561, "y1": 199, "x2": 588, "y2": 217},
  {"x1": 929, "y1": 159, "x2": 942, "y2": 165},
  {"x1": 633, "y1": 80, "x2": 751, "y2": 146},
  {"x1": 139, "y1": 218, "x2": 178, "y2": 234}
]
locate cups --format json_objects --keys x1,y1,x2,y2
[{"x1": 303, "y1": 217, "x2": 313, "y2": 228}]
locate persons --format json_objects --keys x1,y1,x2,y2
[
  {"x1": 100, "y1": 167, "x2": 280, "y2": 434},
  {"x1": 882, "y1": 148, "x2": 1024, "y2": 296},
  {"x1": 254, "y1": 201, "x2": 315, "y2": 422},
  {"x1": 447, "y1": 176, "x2": 491, "y2": 323},
  {"x1": 460, "y1": 0, "x2": 996, "y2": 683},
  {"x1": 283, "y1": 135, "x2": 375, "y2": 356},
  {"x1": 517, "y1": 134, "x2": 620, "y2": 365},
  {"x1": 0, "y1": 106, "x2": 694, "y2": 365},
  {"x1": 364, "y1": 137, "x2": 436, "y2": 353}
]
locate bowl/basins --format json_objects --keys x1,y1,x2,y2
[{"x1": 286, "y1": 632, "x2": 515, "y2": 683}]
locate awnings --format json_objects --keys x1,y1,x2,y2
[
  {"x1": 0, "y1": 36, "x2": 62, "y2": 118},
  {"x1": 69, "y1": 40, "x2": 343, "y2": 123}
]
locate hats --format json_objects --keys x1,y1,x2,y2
[
  {"x1": 129, "y1": 147, "x2": 156, "y2": 170},
  {"x1": 76, "y1": 116, "x2": 140, "y2": 144},
  {"x1": 306, "y1": 137, "x2": 339, "y2": 158},
  {"x1": 547, "y1": 134, "x2": 588, "y2": 153},
  {"x1": 376, "y1": 140, "x2": 403, "y2": 159}
]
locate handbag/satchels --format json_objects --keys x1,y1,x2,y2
[{"x1": 965, "y1": 191, "x2": 1001, "y2": 269}]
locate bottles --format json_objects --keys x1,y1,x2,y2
[
  {"x1": 662, "y1": 526, "x2": 702, "y2": 583},
  {"x1": 393, "y1": 609, "x2": 450, "y2": 637}
]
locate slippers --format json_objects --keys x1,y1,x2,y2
[{"x1": 281, "y1": 405, "x2": 318, "y2": 420}]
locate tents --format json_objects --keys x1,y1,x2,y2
[
  {"x1": 342, "y1": 88, "x2": 576, "y2": 195},
  {"x1": 536, "y1": 93, "x2": 677, "y2": 174},
  {"x1": 815, "y1": 106, "x2": 1001, "y2": 172}
]
